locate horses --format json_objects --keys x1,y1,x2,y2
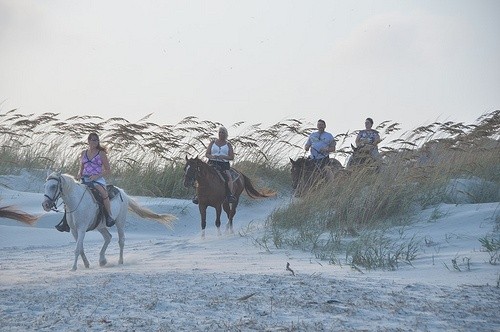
[
  {"x1": 42, "y1": 172, "x2": 178, "y2": 272},
  {"x1": 289, "y1": 156, "x2": 345, "y2": 198},
  {"x1": 346, "y1": 143, "x2": 382, "y2": 179},
  {"x1": 184, "y1": 154, "x2": 277, "y2": 240}
]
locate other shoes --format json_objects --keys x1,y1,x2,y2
[
  {"x1": 105, "y1": 217, "x2": 115, "y2": 227},
  {"x1": 228, "y1": 194, "x2": 234, "y2": 201},
  {"x1": 192, "y1": 198, "x2": 198, "y2": 204},
  {"x1": 56, "y1": 223, "x2": 70, "y2": 232}
]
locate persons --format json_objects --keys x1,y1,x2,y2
[
  {"x1": 192, "y1": 126, "x2": 234, "y2": 204},
  {"x1": 305, "y1": 120, "x2": 335, "y2": 172},
  {"x1": 60, "y1": 132, "x2": 115, "y2": 232},
  {"x1": 356, "y1": 118, "x2": 381, "y2": 161}
]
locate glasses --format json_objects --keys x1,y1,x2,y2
[{"x1": 90, "y1": 139, "x2": 99, "y2": 141}]
